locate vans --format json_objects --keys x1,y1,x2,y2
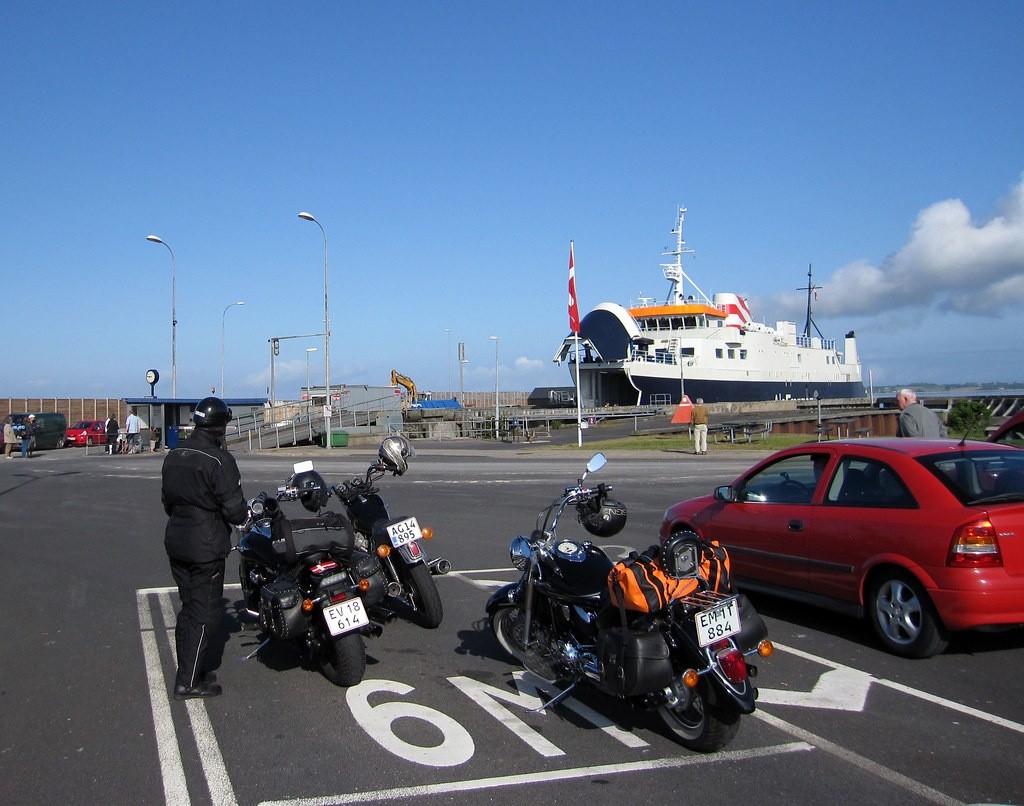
[{"x1": 0, "y1": 411, "x2": 68, "y2": 452}]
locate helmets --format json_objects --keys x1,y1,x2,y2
[
  {"x1": 194, "y1": 397, "x2": 232, "y2": 425},
  {"x1": 293, "y1": 471, "x2": 326, "y2": 512},
  {"x1": 578, "y1": 495, "x2": 626, "y2": 536},
  {"x1": 379, "y1": 437, "x2": 411, "y2": 476}
]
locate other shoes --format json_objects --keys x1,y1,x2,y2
[
  {"x1": 694, "y1": 452, "x2": 699, "y2": 454},
  {"x1": 6, "y1": 456, "x2": 13, "y2": 459},
  {"x1": 702, "y1": 451, "x2": 707, "y2": 455},
  {"x1": 105, "y1": 451, "x2": 109, "y2": 454}
]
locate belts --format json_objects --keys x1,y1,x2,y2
[
  {"x1": 201, "y1": 672, "x2": 217, "y2": 683},
  {"x1": 174, "y1": 682, "x2": 222, "y2": 699}
]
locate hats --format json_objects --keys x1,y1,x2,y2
[{"x1": 29, "y1": 414, "x2": 36, "y2": 418}]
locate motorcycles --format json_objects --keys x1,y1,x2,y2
[
  {"x1": 325, "y1": 458, "x2": 452, "y2": 629},
  {"x1": 484, "y1": 451, "x2": 775, "y2": 754},
  {"x1": 228, "y1": 460, "x2": 398, "y2": 688}
]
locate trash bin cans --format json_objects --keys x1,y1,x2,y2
[
  {"x1": 320, "y1": 430, "x2": 349, "y2": 447},
  {"x1": 167, "y1": 426, "x2": 179, "y2": 448}
]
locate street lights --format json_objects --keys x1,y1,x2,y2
[
  {"x1": 296, "y1": 211, "x2": 332, "y2": 449},
  {"x1": 443, "y1": 328, "x2": 453, "y2": 399},
  {"x1": 488, "y1": 334, "x2": 500, "y2": 442},
  {"x1": 144, "y1": 234, "x2": 178, "y2": 399},
  {"x1": 220, "y1": 301, "x2": 246, "y2": 398},
  {"x1": 306, "y1": 347, "x2": 318, "y2": 400}
]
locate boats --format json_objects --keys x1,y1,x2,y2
[{"x1": 550, "y1": 203, "x2": 869, "y2": 407}]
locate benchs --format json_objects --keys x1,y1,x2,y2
[
  {"x1": 854, "y1": 428, "x2": 872, "y2": 438},
  {"x1": 708, "y1": 428, "x2": 768, "y2": 444},
  {"x1": 815, "y1": 427, "x2": 833, "y2": 442}
]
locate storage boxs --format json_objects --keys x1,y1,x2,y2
[
  {"x1": 342, "y1": 550, "x2": 390, "y2": 608},
  {"x1": 597, "y1": 625, "x2": 673, "y2": 697},
  {"x1": 729, "y1": 594, "x2": 769, "y2": 652},
  {"x1": 259, "y1": 581, "x2": 307, "y2": 651}
]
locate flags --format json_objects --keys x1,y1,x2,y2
[{"x1": 568, "y1": 249, "x2": 580, "y2": 334}]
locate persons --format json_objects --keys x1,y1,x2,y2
[
  {"x1": 161, "y1": 396, "x2": 249, "y2": 701},
  {"x1": 21, "y1": 415, "x2": 37, "y2": 458},
  {"x1": 690, "y1": 399, "x2": 709, "y2": 454},
  {"x1": 4, "y1": 419, "x2": 17, "y2": 459},
  {"x1": 812, "y1": 460, "x2": 825, "y2": 481},
  {"x1": 105, "y1": 414, "x2": 118, "y2": 455},
  {"x1": 896, "y1": 389, "x2": 947, "y2": 438},
  {"x1": 126, "y1": 411, "x2": 140, "y2": 454},
  {"x1": 150, "y1": 426, "x2": 157, "y2": 452}
]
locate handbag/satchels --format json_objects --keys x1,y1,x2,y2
[
  {"x1": 607, "y1": 541, "x2": 731, "y2": 613},
  {"x1": 271, "y1": 515, "x2": 355, "y2": 566}
]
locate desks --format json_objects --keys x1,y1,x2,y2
[
  {"x1": 827, "y1": 419, "x2": 858, "y2": 440},
  {"x1": 722, "y1": 421, "x2": 757, "y2": 444}
]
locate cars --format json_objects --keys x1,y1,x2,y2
[
  {"x1": 64, "y1": 419, "x2": 109, "y2": 448},
  {"x1": 657, "y1": 436, "x2": 1024, "y2": 660},
  {"x1": 946, "y1": 405, "x2": 1024, "y2": 492}
]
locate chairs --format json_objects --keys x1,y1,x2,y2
[{"x1": 837, "y1": 467, "x2": 907, "y2": 505}]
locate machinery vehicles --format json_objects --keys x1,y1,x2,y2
[{"x1": 389, "y1": 368, "x2": 433, "y2": 409}]
